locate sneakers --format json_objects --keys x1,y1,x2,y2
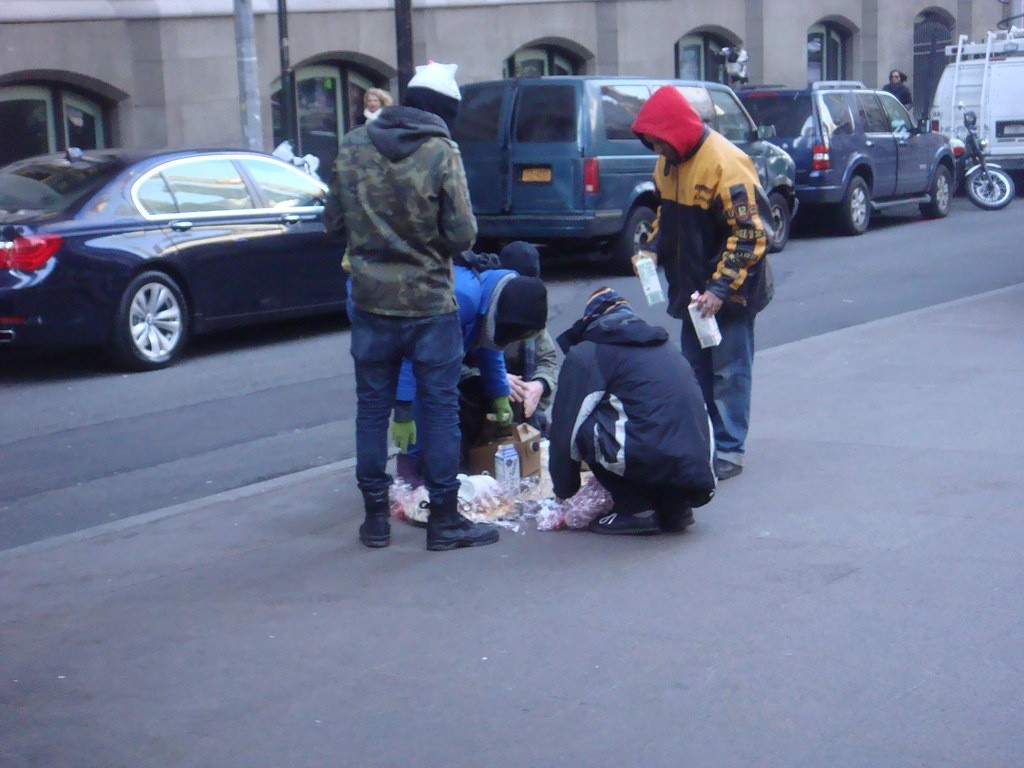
[
  {"x1": 655, "y1": 496, "x2": 695, "y2": 531},
  {"x1": 588, "y1": 508, "x2": 662, "y2": 534}
]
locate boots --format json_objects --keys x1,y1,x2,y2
[
  {"x1": 357, "y1": 482, "x2": 393, "y2": 547},
  {"x1": 420, "y1": 486, "x2": 500, "y2": 551},
  {"x1": 388, "y1": 451, "x2": 427, "y2": 492}
]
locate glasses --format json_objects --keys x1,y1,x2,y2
[{"x1": 891, "y1": 76, "x2": 899, "y2": 79}]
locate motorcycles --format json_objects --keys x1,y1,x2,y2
[{"x1": 960, "y1": 101, "x2": 1016, "y2": 212}]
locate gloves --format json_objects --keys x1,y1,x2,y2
[
  {"x1": 390, "y1": 420, "x2": 417, "y2": 455},
  {"x1": 493, "y1": 395, "x2": 511, "y2": 428}
]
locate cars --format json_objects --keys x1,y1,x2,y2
[{"x1": 0, "y1": 146, "x2": 348, "y2": 371}]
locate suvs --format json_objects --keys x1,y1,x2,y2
[{"x1": 737, "y1": 79, "x2": 960, "y2": 234}]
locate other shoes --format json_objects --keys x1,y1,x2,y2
[{"x1": 716, "y1": 458, "x2": 743, "y2": 479}]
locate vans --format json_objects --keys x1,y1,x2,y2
[
  {"x1": 456, "y1": 74, "x2": 799, "y2": 273},
  {"x1": 927, "y1": 25, "x2": 1024, "y2": 171}
]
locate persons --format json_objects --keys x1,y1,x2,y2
[
  {"x1": 548, "y1": 286, "x2": 716, "y2": 534},
  {"x1": 335, "y1": 62, "x2": 564, "y2": 550},
  {"x1": 882, "y1": 70, "x2": 912, "y2": 111},
  {"x1": 631, "y1": 85, "x2": 774, "y2": 480}
]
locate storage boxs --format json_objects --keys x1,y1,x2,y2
[{"x1": 473, "y1": 422, "x2": 544, "y2": 481}]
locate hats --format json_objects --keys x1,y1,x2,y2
[
  {"x1": 582, "y1": 285, "x2": 627, "y2": 322},
  {"x1": 405, "y1": 61, "x2": 461, "y2": 117},
  {"x1": 500, "y1": 240, "x2": 541, "y2": 277}
]
